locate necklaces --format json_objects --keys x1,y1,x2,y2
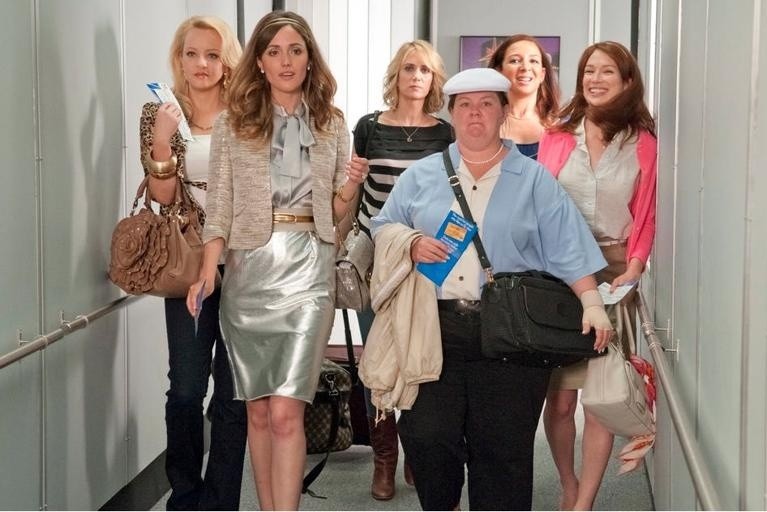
[
  {"x1": 587, "y1": 118, "x2": 610, "y2": 149},
  {"x1": 189, "y1": 121, "x2": 213, "y2": 132},
  {"x1": 507, "y1": 113, "x2": 527, "y2": 121},
  {"x1": 459, "y1": 144, "x2": 505, "y2": 165},
  {"x1": 397, "y1": 112, "x2": 422, "y2": 143}
]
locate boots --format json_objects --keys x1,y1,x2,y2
[
  {"x1": 370, "y1": 410, "x2": 398, "y2": 500},
  {"x1": 401, "y1": 409, "x2": 415, "y2": 488}
]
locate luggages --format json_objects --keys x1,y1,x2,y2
[{"x1": 338, "y1": 308, "x2": 372, "y2": 446}]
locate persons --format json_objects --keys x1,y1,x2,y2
[
  {"x1": 139, "y1": 15, "x2": 247, "y2": 511},
  {"x1": 185, "y1": 10, "x2": 369, "y2": 511},
  {"x1": 484, "y1": 33, "x2": 571, "y2": 166},
  {"x1": 369, "y1": 68, "x2": 614, "y2": 510},
  {"x1": 536, "y1": 41, "x2": 658, "y2": 510},
  {"x1": 348, "y1": 38, "x2": 456, "y2": 500}
]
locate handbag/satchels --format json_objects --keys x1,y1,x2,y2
[
  {"x1": 332, "y1": 190, "x2": 374, "y2": 313},
  {"x1": 480, "y1": 270, "x2": 607, "y2": 367},
  {"x1": 304, "y1": 357, "x2": 352, "y2": 454},
  {"x1": 108, "y1": 174, "x2": 222, "y2": 299},
  {"x1": 581, "y1": 304, "x2": 656, "y2": 438}
]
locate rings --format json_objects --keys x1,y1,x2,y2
[{"x1": 361, "y1": 172, "x2": 368, "y2": 180}]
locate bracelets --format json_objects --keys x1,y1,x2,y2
[
  {"x1": 145, "y1": 148, "x2": 178, "y2": 179},
  {"x1": 337, "y1": 185, "x2": 357, "y2": 203}
]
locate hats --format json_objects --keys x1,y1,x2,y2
[{"x1": 444, "y1": 68, "x2": 512, "y2": 96}]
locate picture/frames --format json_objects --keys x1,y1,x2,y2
[{"x1": 458, "y1": 36, "x2": 560, "y2": 104}]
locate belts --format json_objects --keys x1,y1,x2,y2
[
  {"x1": 272, "y1": 212, "x2": 313, "y2": 224},
  {"x1": 436, "y1": 298, "x2": 480, "y2": 316},
  {"x1": 597, "y1": 240, "x2": 627, "y2": 247}
]
